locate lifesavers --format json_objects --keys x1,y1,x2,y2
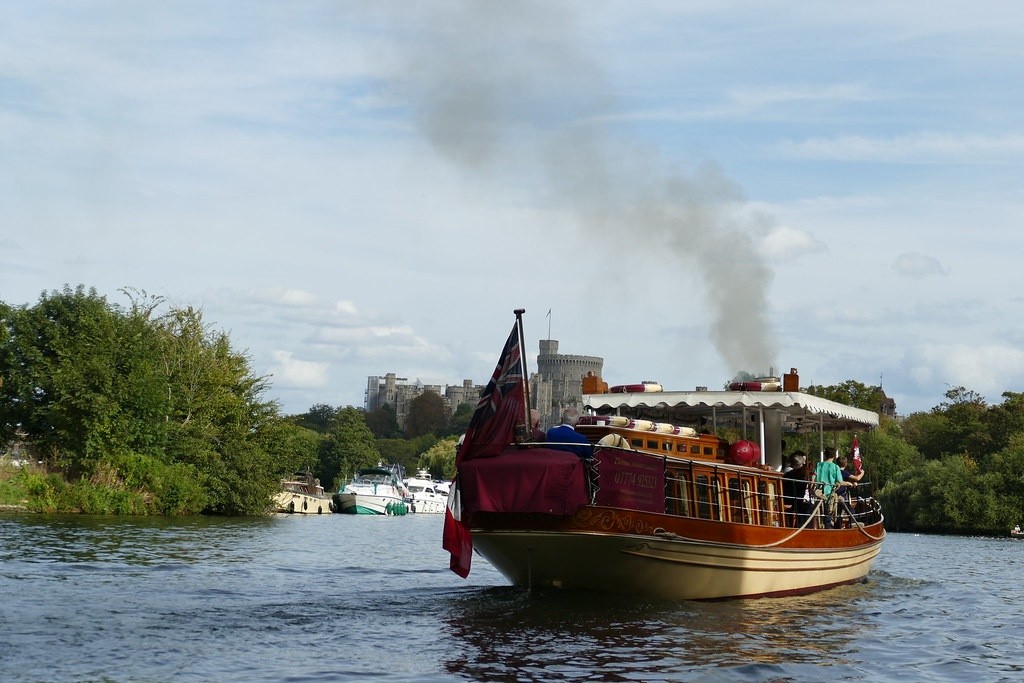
[
  {"x1": 610, "y1": 382, "x2": 663, "y2": 394},
  {"x1": 578, "y1": 416, "x2": 630, "y2": 427},
  {"x1": 627, "y1": 418, "x2": 697, "y2": 436},
  {"x1": 728, "y1": 381, "x2": 781, "y2": 392},
  {"x1": 592, "y1": 434, "x2": 632, "y2": 484}
]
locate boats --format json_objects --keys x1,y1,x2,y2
[
  {"x1": 269, "y1": 480, "x2": 334, "y2": 514},
  {"x1": 1010, "y1": 529, "x2": 1024, "y2": 539},
  {"x1": 459, "y1": 308, "x2": 889, "y2": 603},
  {"x1": 331, "y1": 457, "x2": 452, "y2": 516}
]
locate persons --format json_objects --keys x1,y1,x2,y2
[
  {"x1": 781, "y1": 438, "x2": 865, "y2": 529},
  {"x1": 529, "y1": 408, "x2": 547, "y2": 450},
  {"x1": 547, "y1": 408, "x2": 593, "y2": 460}
]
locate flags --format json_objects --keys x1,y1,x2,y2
[
  {"x1": 852, "y1": 434, "x2": 862, "y2": 476},
  {"x1": 442, "y1": 319, "x2": 525, "y2": 580}
]
